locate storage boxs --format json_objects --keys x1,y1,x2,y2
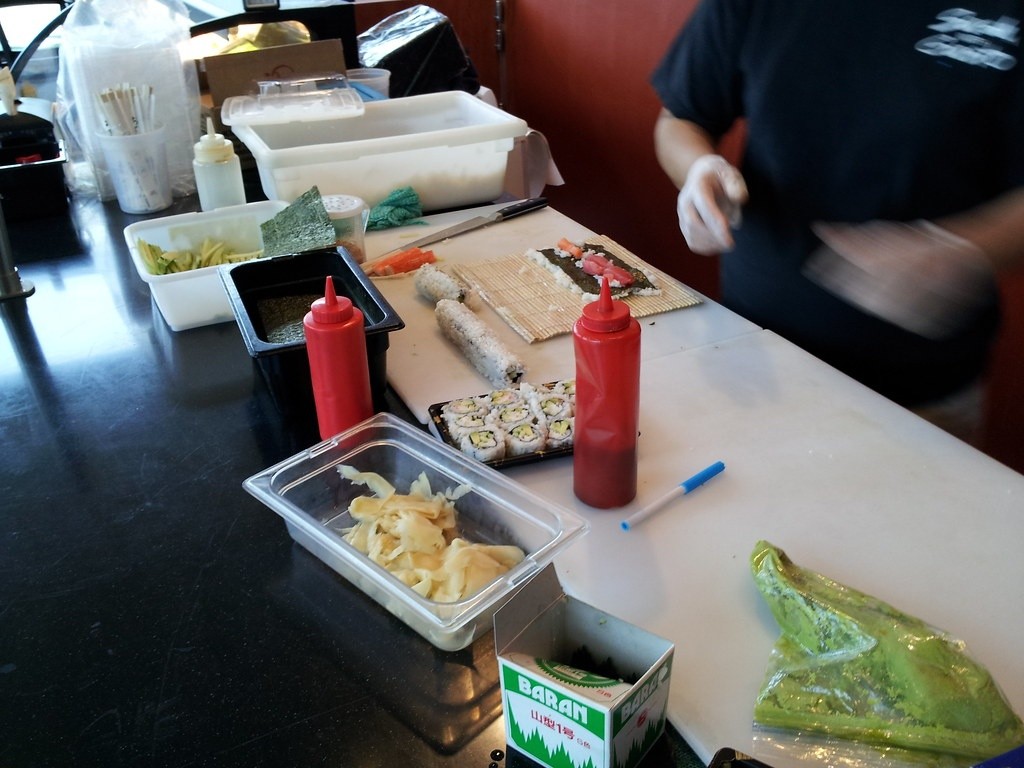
[
  {"x1": 492, "y1": 561, "x2": 675, "y2": 768},
  {"x1": 230, "y1": 90, "x2": 527, "y2": 212},
  {"x1": 200, "y1": 39, "x2": 347, "y2": 138},
  {"x1": 123, "y1": 201, "x2": 294, "y2": 331}
]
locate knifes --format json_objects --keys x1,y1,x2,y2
[{"x1": 388, "y1": 196, "x2": 550, "y2": 257}]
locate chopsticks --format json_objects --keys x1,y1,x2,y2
[{"x1": 99, "y1": 82, "x2": 166, "y2": 211}]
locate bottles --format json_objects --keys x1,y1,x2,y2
[
  {"x1": 193, "y1": 117, "x2": 247, "y2": 212},
  {"x1": 303, "y1": 276, "x2": 377, "y2": 450},
  {"x1": 572, "y1": 275, "x2": 642, "y2": 509}
]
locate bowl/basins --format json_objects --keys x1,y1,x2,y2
[
  {"x1": 216, "y1": 243, "x2": 404, "y2": 409},
  {"x1": 124, "y1": 200, "x2": 317, "y2": 331},
  {"x1": 242, "y1": 413, "x2": 591, "y2": 654}
]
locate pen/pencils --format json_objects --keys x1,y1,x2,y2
[{"x1": 621, "y1": 460, "x2": 726, "y2": 531}]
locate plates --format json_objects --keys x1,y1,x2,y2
[{"x1": 433, "y1": 383, "x2": 599, "y2": 466}]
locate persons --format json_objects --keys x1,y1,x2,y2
[{"x1": 649, "y1": 0, "x2": 1024, "y2": 447}]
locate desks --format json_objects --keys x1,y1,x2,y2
[{"x1": 359, "y1": 198, "x2": 1024, "y2": 768}]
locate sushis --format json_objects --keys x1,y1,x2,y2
[
  {"x1": 439, "y1": 377, "x2": 576, "y2": 462},
  {"x1": 412, "y1": 263, "x2": 526, "y2": 390}
]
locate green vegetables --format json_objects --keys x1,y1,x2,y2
[
  {"x1": 744, "y1": 539, "x2": 1024, "y2": 768},
  {"x1": 138, "y1": 238, "x2": 230, "y2": 275}
]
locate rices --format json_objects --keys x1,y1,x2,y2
[{"x1": 524, "y1": 240, "x2": 664, "y2": 302}]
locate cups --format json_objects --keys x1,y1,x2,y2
[
  {"x1": 345, "y1": 68, "x2": 392, "y2": 97},
  {"x1": 96, "y1": 123, "x2": 174, "y2": 215}
]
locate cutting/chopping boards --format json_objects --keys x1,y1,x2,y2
[{"x1": 364, "y1": 199, "x2": 764, "y2": 426}]
